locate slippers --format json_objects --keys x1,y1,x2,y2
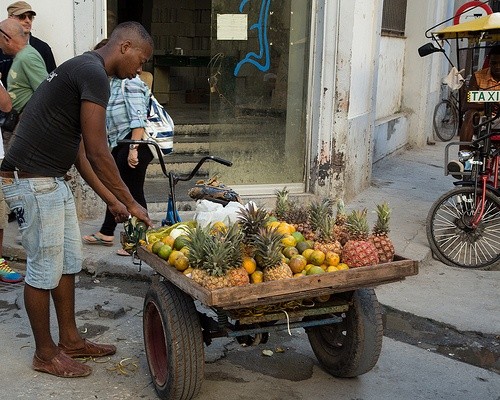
[
  {"x1": 82, "y1": 233, "x2": 112, "y2": 245},
  {"x1": 116, "y1": 247, "x2": 131, "y2": 255}
]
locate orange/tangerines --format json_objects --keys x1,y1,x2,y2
[{"x1": 139, "y1": 216, "x2": 349, "y2": 283}]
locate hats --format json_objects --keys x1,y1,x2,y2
[{"x1": 7, "y1": 1, "x2": 36, "y2": 18}]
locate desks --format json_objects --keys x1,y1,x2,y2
[{"x1": 150, "y1": 55, "x2": 217, "y2": 105}]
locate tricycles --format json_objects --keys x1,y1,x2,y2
[{"x1": 417, "y1": 10, "x2": 500, "y2": 271}]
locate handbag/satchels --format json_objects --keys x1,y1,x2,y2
[
  {"x1": 0, "y1": 109, "x2": 20, "y2": 133},
  {"x1": 120, "y1": 78, "x2": 175, "y2": 158}
]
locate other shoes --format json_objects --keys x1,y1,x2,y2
[
  {"x1": 32, "y1": 348, "x2": 93, "y2": 378},
  {"x1": 449, "y1": 160, "x2": 464, "y2": 180},
  {"x1": 58, "y1": 338, "x2": 116, "y2": 358}
]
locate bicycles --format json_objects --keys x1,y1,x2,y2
[{"x1": 434, "y1": 81, "x2": 461, "y2": 143}]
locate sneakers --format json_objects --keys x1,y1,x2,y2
[{"x1": 0, "y1": 258, "x2": 23, "y2": 282}]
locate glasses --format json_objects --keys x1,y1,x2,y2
[{"x1": 10, "y1": 14, "x2": 34, "y2": 21}]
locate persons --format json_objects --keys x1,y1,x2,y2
[
  {"x1": 0, "y1": 21, "x2": 154, "y2": 377},
  {"x1": 0, "y1": 80, "x2": 23, "y2": 282},
  {"x1": 0, "y1": 0, "x2": 57, "y2": 79},
  {"x1": 82, "y1": 39, "x2": 154, "y2": 255},
  {"x1": 460, "y1": 43, "x2": 500, "y2": 151},
  {"x1": 0, "y1": 19, "x2": 48, "y2": 242}
]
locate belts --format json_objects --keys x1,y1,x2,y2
[{"x1": 0, "y1": 169, "x2": 72, "y2": 182}]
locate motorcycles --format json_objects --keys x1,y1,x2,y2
[{"x1": 115, "y1": 137, "x2": 386, "y2": 400}]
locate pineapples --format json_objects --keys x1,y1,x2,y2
[{"x1": 190, "y1": 189, "x2": 395, "y2": 291}]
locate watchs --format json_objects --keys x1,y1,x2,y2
[{"x1": 129, "y1": 144, "x2": 139, "y2": 149}]
